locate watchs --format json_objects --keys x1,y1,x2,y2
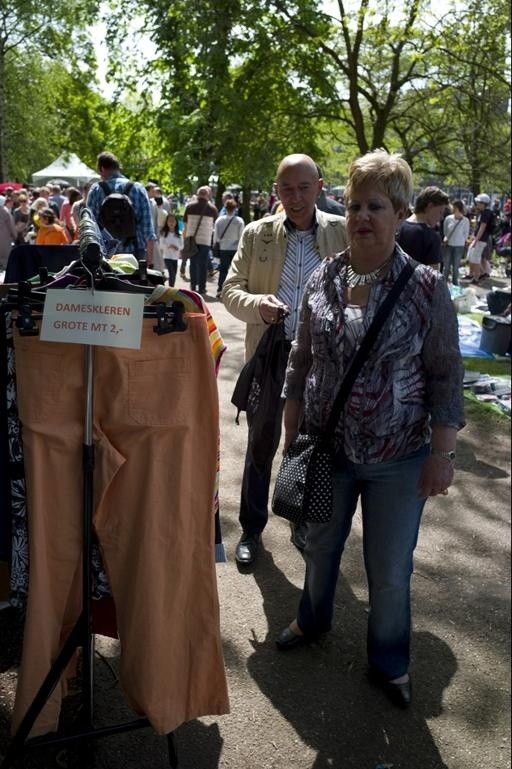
[{"x1": 430, "y1": 448, "x2": 458, "y2": 463}]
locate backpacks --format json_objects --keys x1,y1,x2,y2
[{"x1": 97, "y1": 181, "x2": 137, "y2": 247}]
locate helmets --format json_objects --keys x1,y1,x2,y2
[{"x1": 475, "y1": 194, "x2": 490, "y2": 204}]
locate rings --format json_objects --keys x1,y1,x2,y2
[{"x1": 271, "y1": 317, "x2": 276, "y2": 322}]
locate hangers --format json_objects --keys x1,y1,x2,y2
[{"x1": 7, "y1": 207, "x2": 186, "y2": 337}]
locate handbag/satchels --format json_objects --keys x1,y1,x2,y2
[
  {"x1": 232, "y1": 326, "x2": 289, "y2": 427},
  {"x1": 213, "y1": 243, "x2": 220, "y2": 257},
  {"x1": 181, "y1": 236, "x2": 200, "y2": 259},
  {"x1": 272, "y1": 432, "x2": 335, "y2": 526}
]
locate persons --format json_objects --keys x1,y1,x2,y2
[
  {"x1": 87, "y1": 150, "x2": 158, "y2": 270},
  {"x1": 314, "y1": 164, "x2": 346, "y2": 217},
  {"x1": 144, "y1": 182, "x2": 284, "y2": 297},
  {"x1": 396, "y1": 186, "x2": 512, "y2": 287},
  {"x1": 0, "y1": 183, "x2": 83, "y2": 271},
  {"x1": 219, "y1": 151, "x2": 350, "y2": 566},
  {"x1": 271, "y1": 146, "x2": 469, "y2": 709}
]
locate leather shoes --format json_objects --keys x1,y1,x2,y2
[
  {"x1": 386, "y1": 672, "x2": 412, "y2": 709},
  {"x1": 292, "y1": 523, "x2": 308, "y2": 549},
  {"x1": 235, "y1": 531, "x2": 261, "y2": 567},
  {"x1": 275, "y1": 626, "x2": 304, "y2": 649}
]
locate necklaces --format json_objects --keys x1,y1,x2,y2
[{"x1": 346, "y1": 259, "x2": 390, "y2": 288}]
